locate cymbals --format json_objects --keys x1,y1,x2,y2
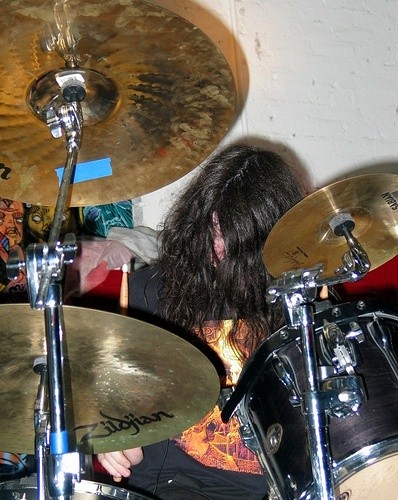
[
  {"x1": 0, "y1": 0, "x2": 249, "y2": 208},
  {"x1": 260, "y1": 171, "x2": 398, "y2": 287},
  {"x1": 0, "y1": 302, "x2": 219, "y2": 456}
]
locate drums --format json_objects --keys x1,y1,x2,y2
[
  {"x1": 233, "y1": 303, "x2": 398, "y2": 500},
  {"x1": 0, "y1": 476, "x2": 148, "y2": 500}
]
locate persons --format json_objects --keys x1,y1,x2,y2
[{"x1": 96, "y1": 144, "x2": 307, "y2": 500}]
